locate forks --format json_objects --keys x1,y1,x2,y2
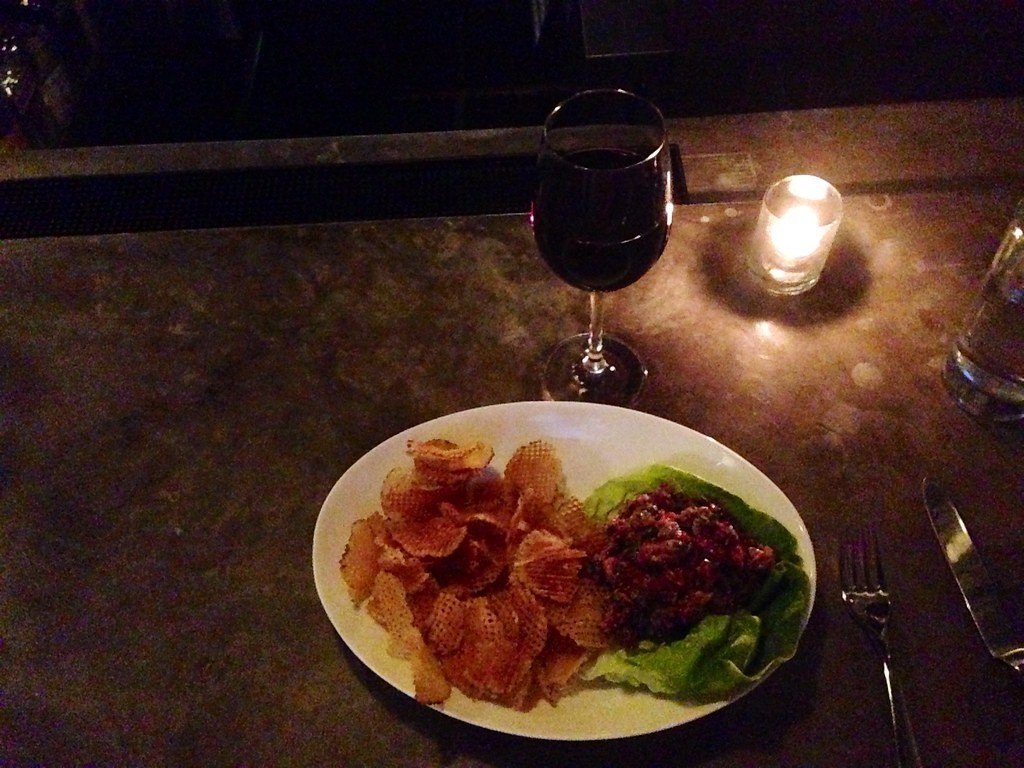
[{"x1": 839, "y1": 526, "x2": 924, "y2": 768}]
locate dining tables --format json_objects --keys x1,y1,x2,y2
[{"x1": 0, "y1": 98, "x2": 1024, "y2": 768}]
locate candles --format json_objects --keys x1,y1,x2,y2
[{"x1": 747, "y1": 174, "x2": 843, "y2": 298}]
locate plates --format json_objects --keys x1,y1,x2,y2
[{"x1": 311, "y1": 401, "x2": 818, "y2": 742}]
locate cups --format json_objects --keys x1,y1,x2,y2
[
  {"x1": 748, "y1": 172, "x2": 843, "y2": 296},
  {"x1": 943, "y1": 213, "x2": 1024, "y2": 429}
]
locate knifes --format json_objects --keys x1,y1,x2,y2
[{"x1": 921, "y1": 476, "x2": 1024, "y2": 675}]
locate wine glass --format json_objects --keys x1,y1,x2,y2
[{"x1": 535, "y1": 88, "x2": 672, "y2": 407}]
